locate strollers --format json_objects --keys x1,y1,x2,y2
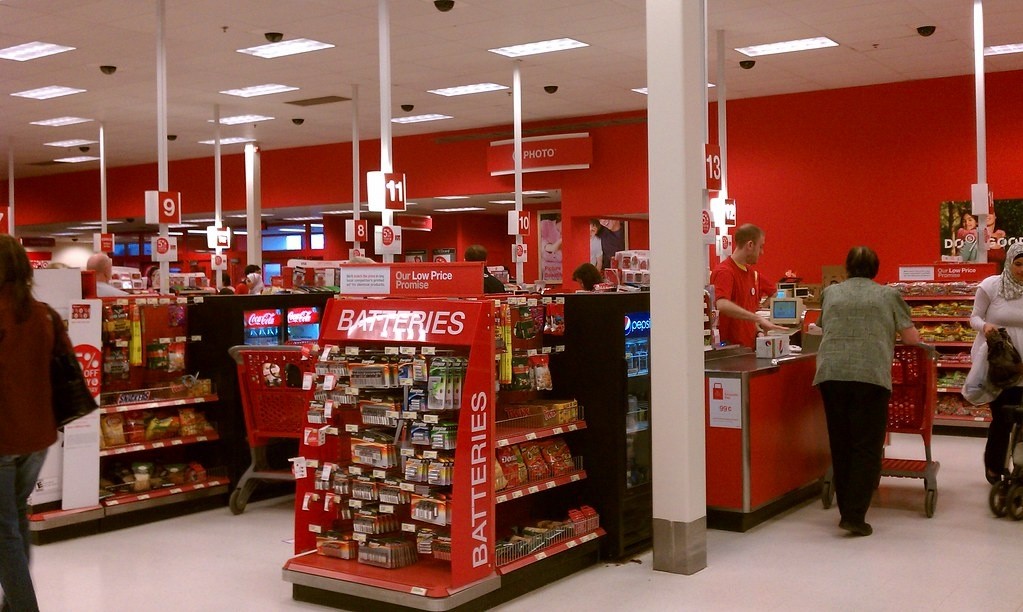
[{"x1": 987, "y1": 401, "x2": 1023, "y2": 522}]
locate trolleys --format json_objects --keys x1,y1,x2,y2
[
  {"x1": 819, "y1": 339, "x2": 943, "y2": 519},
  {"x1": 224, "y1": 343, "x2": 315, "y2": 517}
]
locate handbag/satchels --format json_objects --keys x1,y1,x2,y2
[
  {"x1": 985, "y1": 328, "x2": 1023, "y2": 388},
  {"x1": 961, "y1": 342, "x2": 1003, "y2": 407},
  {"x1": 41, "y1": 301, "x2": 99, "y2": 429}
]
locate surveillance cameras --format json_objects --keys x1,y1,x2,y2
[
  {"x1": 739, "y1": 60, "x2": 755, "y2": 69},
  {"x1": 543, "y1": 86, "x2": 558, "y2": 94},
  {"x1": 167, "y1": 135, "x2": 177, "y2": 141},
  {"x1": 434, "y1": 0, "x2": 454, "y2": 12},
  {"x1": 265, "y1": 32, "x2": 283, "y2": 43},
  {"x1": 79, "y1": 147, "x2": 90, "y2": 152},
  {"x1": 99, "y1": 66, "x2": 116, "y2": 75},
  {"x1": 292, "y1": 119, "x2": 304, "y2": 125},
  {"x1": 401, "y1": 105, "x2": 413, "y2": 111},
  {"x1": 918, "y1": 26, "x2": 935, "y2": 37}
]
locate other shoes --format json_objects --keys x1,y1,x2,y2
[
  {"x1": 985, "y1": 464, "x2": 1002, "y2": 485},
  {"x1": 839, "y1": 518, "x2": 873, "y2": 536}
]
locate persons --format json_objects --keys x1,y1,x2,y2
[
  {"x1": 970, "y1": 243, "x2": 1023, "y2": 484},
  {"x1": 813, "y1": 246, "x2": 923, "y2": 538},
  {"x1": 144, "y1": 265, "x2": 160, "y2": 291},
  {"x1": 0, "y1": 232, "x2": 58, "y2": 612},
  {"x1": 590, "y1": 217, "x2": 626, "y2": 271},
  {"x1": 45, "y1": 253, "x2": 131, "y2": 297},
  {"x1": 213, "y1": 264, "x2": 266, "y2": 294},
  {"x1": 709, "y1": 223, "x2": 791, "y2": 349},
  {"x1": 462, "y1": 244, "x2": 506, "y2": 293},
  {"x1": 571, "y1": 263, "x2": 604, "y2": 291},
  {"x1": 956, "y1": 209, "x2": 1008, "y2": 262}
]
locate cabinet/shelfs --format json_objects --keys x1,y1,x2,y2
[
  {"x1": 60, "y1": 291, "x2": 605, "y2": 612},
  {"x1": 901, "y1": 292, "x2": 992, "y2": 430}
]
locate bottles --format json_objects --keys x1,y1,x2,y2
[{"x1": 624, "y1": 338, "x2": 652, "y2": 489}]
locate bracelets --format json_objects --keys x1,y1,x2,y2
[{"x1": 757, "y1": 331, "x2": 764, "y2": 335}]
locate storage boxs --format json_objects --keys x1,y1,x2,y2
[{"x1": 756, "y1": 335, "x2": 791, "y2": 359}]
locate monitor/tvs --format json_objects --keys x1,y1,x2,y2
[
  {"x1": 770, "y1": 298, "x2": 803, "y2": 325},
  {"x1": 777, "y1": 289, "x2": 791, "y2": 298}
]
